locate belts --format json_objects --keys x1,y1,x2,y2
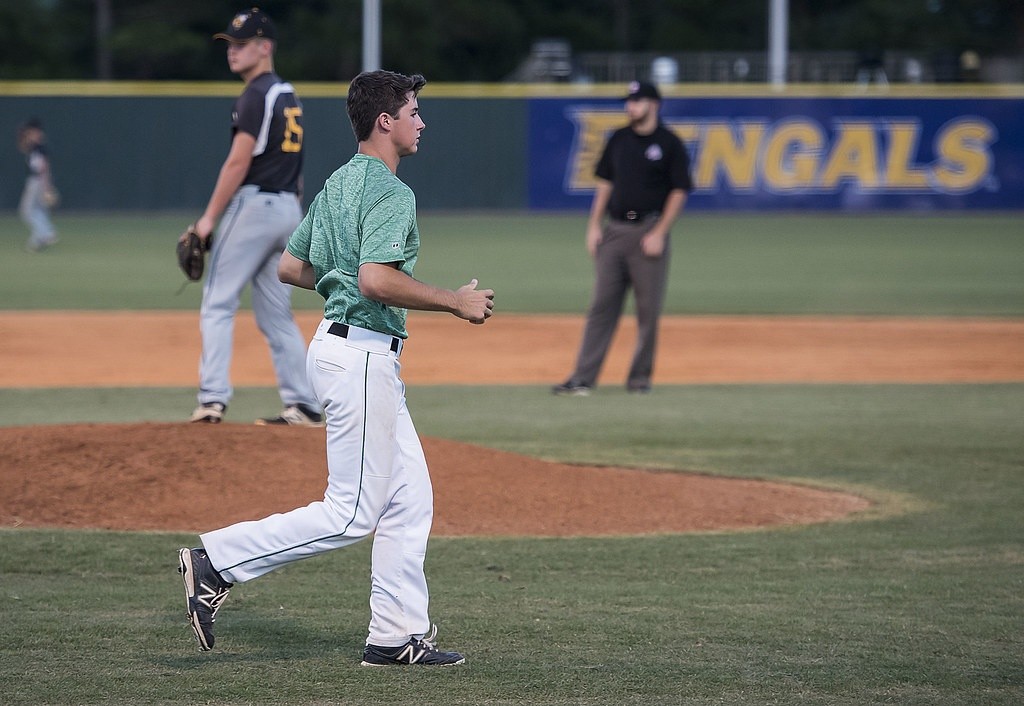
[
  {"x1": 259, "y1": 185, "x2": 280, "y2": 194},
  {"x1": 613, "y1": 211, "x2": 652, "y2": 223},
  {"x1": 328, "y1": 322, "x2": 399, "y2": 352}
]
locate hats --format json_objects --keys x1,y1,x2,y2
[
  {"x1": 211, "y1": 8, "x2": 282, "y2": 44},
  {"x1": 620, "y1": 82, "x2": 660, "y2": 101}
]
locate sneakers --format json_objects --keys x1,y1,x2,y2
[
  {"x1": 255, "y1": 405, "x2": 326, "y2": 428},
  {"x1": 178, "y1": 547, "x2": 234, "y2": 651},
  {"x1": 362, "y1": 625, "x2": 465, "y2": 668},
  {"x1": 192, "y1": 402, "x2": 225, "y2": 423}
]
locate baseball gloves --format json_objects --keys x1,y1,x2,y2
[{"x1": 176, "y1": 222, "x2": 215, "y2": 283}]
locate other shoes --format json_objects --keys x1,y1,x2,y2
[{"x1": 554, "y1": 378, "x2": 591, "y2": 398}]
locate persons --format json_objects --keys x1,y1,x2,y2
[
  {"x1": 12, "y1": 116, "x2": 62, "y2": 254},
  {"x1": 854, "y1": 32, "x2": 888, "y2": 85},
  {"x1": 177, "y1": 6, "x2": 326, "y2": 429},
  {"x1": 175, "y1": 68, "x2": 496, "y2": 671},
  {"x1": 549, "y1": 79, "x2": 693, "y2": 399}
]
